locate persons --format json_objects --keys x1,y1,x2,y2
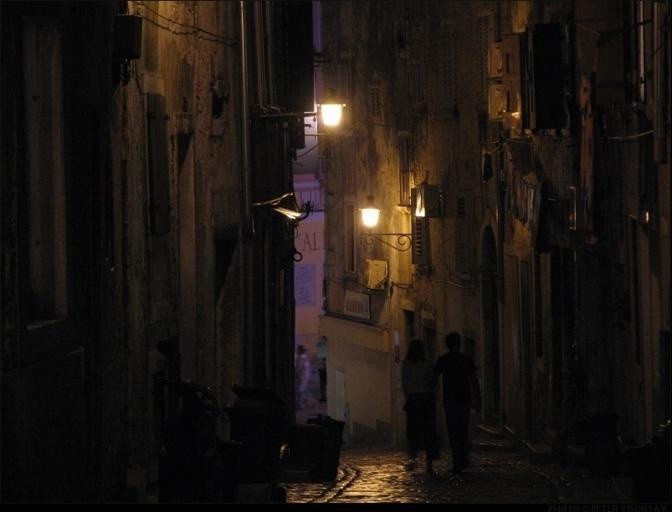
[
  {"x1": 398, "y1": 334, "x2": 441, "y2": 478},
  {"x1": 315, "y1": 335, "x2": 328, "y2": 403},
  {"x1": 435, "y1": 330, "x2": 483, "y2": 473},
  {"x1": 295, "y1": 343, "x2": 313, "y2": 411}
]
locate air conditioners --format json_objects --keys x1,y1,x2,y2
[{"x1": 410, "y1": 187, "x2": 443, "y2": 219}]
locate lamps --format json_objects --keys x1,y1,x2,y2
[
  {"x1": 251, "y1": 193, "x2": 301, "y2": 220},
  {"x1": 304, "y1": 88, "x2": 346, "y2": 126},
  {"x1": 357, "y1": 196, "x2": 411, "y2": 253}
]
